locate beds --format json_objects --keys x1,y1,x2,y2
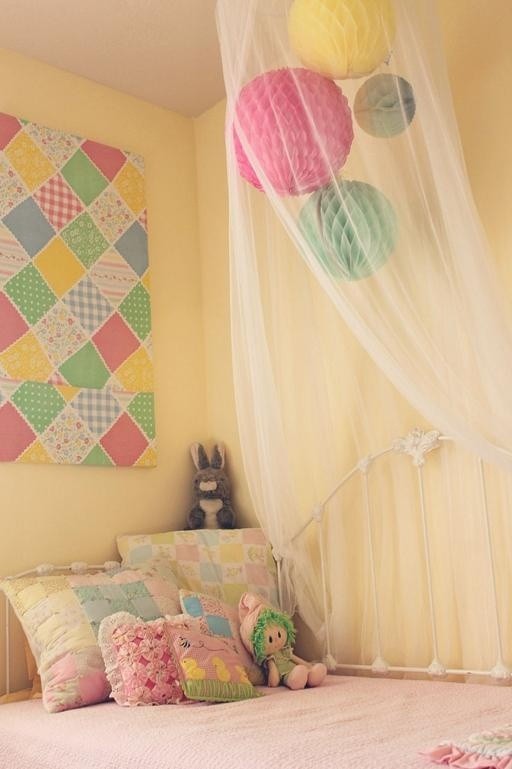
[{"x1": 0, "y1": 560, "x2": 511, "y2": 769}]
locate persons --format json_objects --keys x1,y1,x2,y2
[{"x1": 239, "y1": 591, "x2": 337, "y2": 689}]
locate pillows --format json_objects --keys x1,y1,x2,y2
[
  {"x1": 1, "y1": 570, "x2": 179, "y2": 711},
  {"x1": 113, "y1": 528, "x2": 282, "y2": 612},
  {"x1": 97, "y1": 589, "x2": 265, "y2": 711}
]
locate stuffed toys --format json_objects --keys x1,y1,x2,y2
[{"x1": 187, "y1": 441, "x2": 236, "y2": 528}]
